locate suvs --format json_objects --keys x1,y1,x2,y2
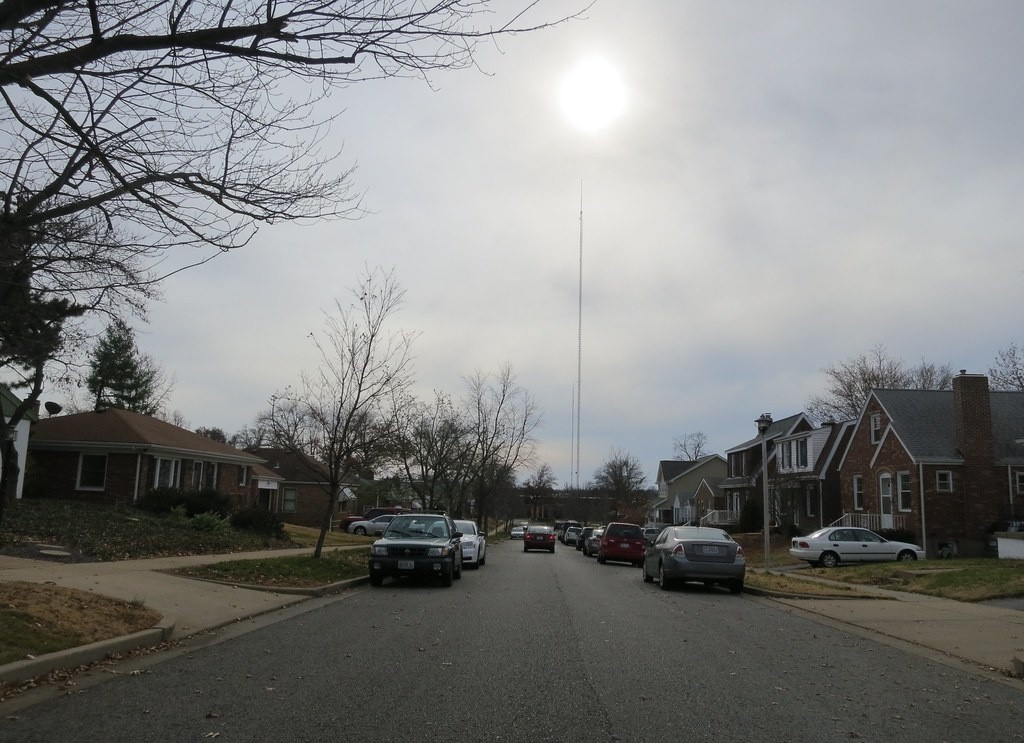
[
  {"x1": 341, "y1": 506, "x2": 414, "y2": 533},
  {"x1": 368, "y1": 510, "x2": 463, "y2": 588}
]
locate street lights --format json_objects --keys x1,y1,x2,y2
[{"x1": 754, "y1": 412, "x2": 774, "y2": 574}]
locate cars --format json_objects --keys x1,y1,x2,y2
[
  {"x1": 519, "y1": 521, "x2": 529, "y2": 529},
  {"x1": 348, "y1": 515, "x2": 427, "y2": 537},
  {"x1": 583, "y1": 529, "x2": 606, "y2": 558},
  {"x1": 643, "y1": 526, "x2": 747, "y2": 595},
  {"x1": 510, "y1": 526, "x2": 524, "y2": 538},
  {"x1": 424, "y1": 519, "x2": 487, "y2": 569},
  {"x1": 596, "y1": 522, "x2": 645, "y2": 567},
  {"x1": 640, "y1": 527, "x2": 662, "y2": 544},
  {"x1": 523, "y1": 525, "x2": 556, "y2": 553},
  {"x1": 789, "y1": 526, "x2": 927, "y2": 568},
  {"x1": 554, "y1": 518, "x2": 608, "y2": 551}
]
